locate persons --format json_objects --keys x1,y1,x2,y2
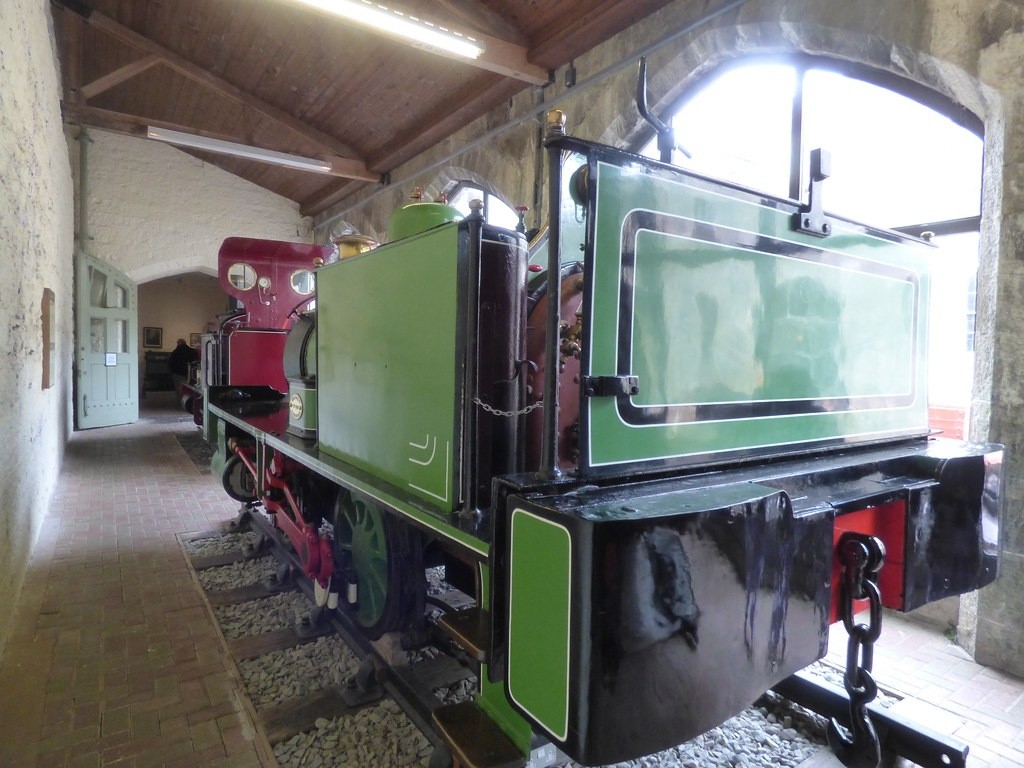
[{"x1": 169, "y1": 338, "x2": 198, "y2": 398}]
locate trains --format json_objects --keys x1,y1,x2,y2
[{"x1": 180, "y1": 57, "x2": 1001, "y2": 768}]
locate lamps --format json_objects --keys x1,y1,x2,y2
[
  {"x1": 304, "y1": 0, "x2": 485, "y2": 61},
  {"x1": 147, "y1": 126, "x2": 332, "y2": 174}
]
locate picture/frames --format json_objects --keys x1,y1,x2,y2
[
  {"x1": 190, "y1": 332, "x2": 201, "y2": 347},
  {"x1": 143, "y1": 327, "x2": 162, "y2": 348}
]
